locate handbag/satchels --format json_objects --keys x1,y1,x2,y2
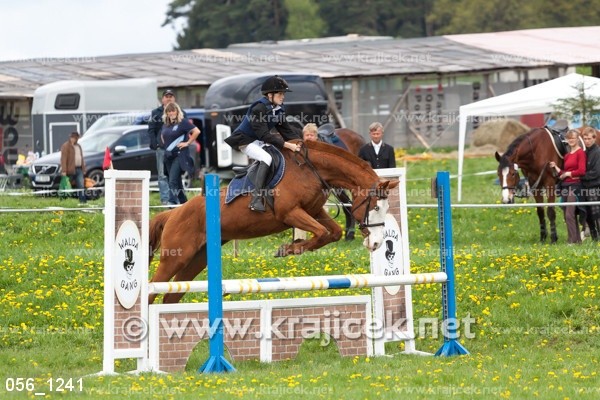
[{"x1": 59, "y1": 175, "x2": 71, "y2": 196}]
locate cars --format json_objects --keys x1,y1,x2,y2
[{"x1": 25, "y1": 107, "x2": 205, "y2": 200}]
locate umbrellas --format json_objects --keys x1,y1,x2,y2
[{"x1": 458, "y1": 72, "x2": 599, "y2": 203}]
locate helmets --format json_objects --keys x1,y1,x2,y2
[{"x1": 261, "y1": 75, "x2": 292, "y2": 95}]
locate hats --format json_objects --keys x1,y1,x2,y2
[
  {"x1": 123, "y1": 249, "x2": 135, "y2": 270},
  {"x1": 386, "y1": 240, "x2": 395, "y2": 260},
  {"x1": 69, "y1": 132, "x2": 80, "y2": 137},
  {"x1": 162, "y1": 88, "x2": 175, "y2": 97}
]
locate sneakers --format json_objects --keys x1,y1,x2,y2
[{"x1": 163, "y1": 201, "x2": 180, "y2": 209}]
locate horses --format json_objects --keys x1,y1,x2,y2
[
  {"x1": 270, "y1": 125, "x2": 365, "y2": 240},
  {"x1": 495, "y1": 126, "x2": 600, "y2": 244},
  {"x1": 146, "y1": 138, "x2": 401, "y2": 305}
]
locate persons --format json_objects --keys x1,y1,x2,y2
[
  {"x1": 61, "y1": 132, "x2": 87, "y2": 203},
  {"x1": 572, "y1": 127, "x2": 600, "y2": 241},
  {"x1": 224, "y1": 74, "x2": 301, "y2": 213},
  {"x1": 359, "y1": 122, "x2": 396, "y2": 169},
  {"x1": 160, "y1": 102, "x2": 201, "y2": 209},
  {"x1": 549, "y1": 129, "x2": 586, "y2": 244},
  {"x1": 301, "y1": 122, "x2": 319, "y2": 142},
  {"x1": 147, "y1": 89, "x2": 190, "y2": 204}
]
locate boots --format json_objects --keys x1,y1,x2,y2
[{"x1": 248, "y1": 161, "x2": 270, "y2": 211}]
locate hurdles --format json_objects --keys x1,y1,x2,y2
[
  {"x1": 198, "y1": 170, "x2": 472, "y2": 374},
  {"x1": 101, "y1": 167, "x2": 434, "y2": 375}
]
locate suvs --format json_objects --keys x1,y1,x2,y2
[{"x1": 82, "y1": 107, "x2": 205, "y2": 160}]
